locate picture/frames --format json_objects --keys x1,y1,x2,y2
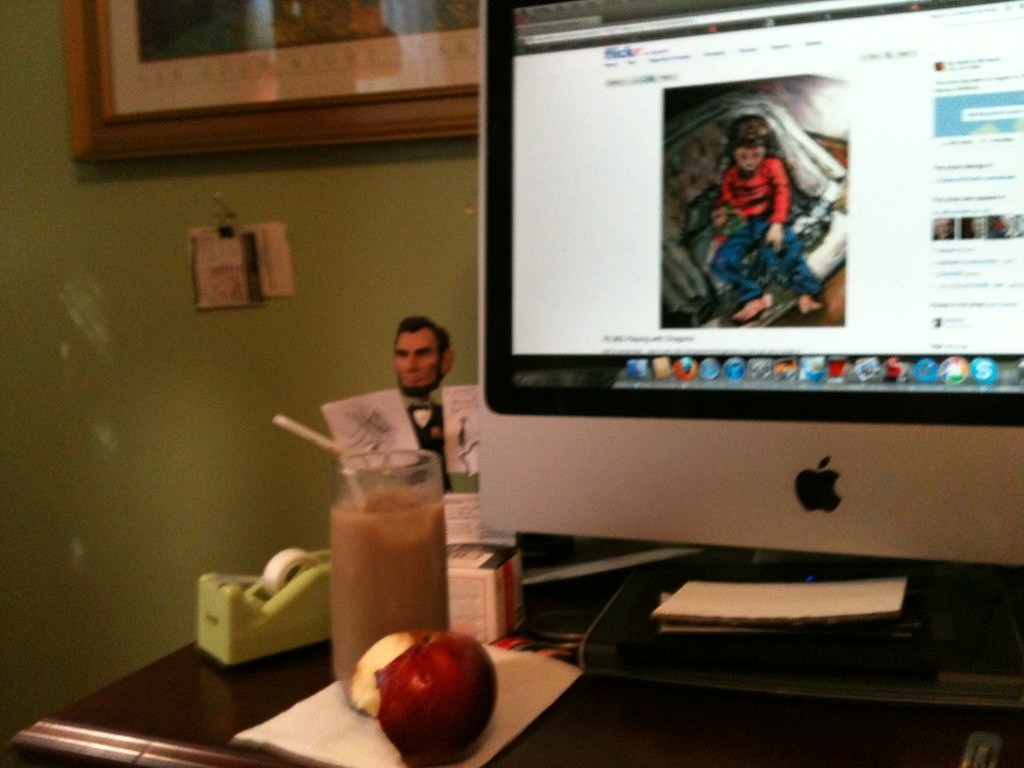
[{"x1": 63, "y1": 0, "x2": 479, "y2": 162}]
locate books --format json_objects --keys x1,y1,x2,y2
[{"x1": 652, "y1": 578, "x2": 908, "y2": 625}]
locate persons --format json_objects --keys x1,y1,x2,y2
[{"x1": 394, "y1": 316, "x2": 454, "y2": 492}]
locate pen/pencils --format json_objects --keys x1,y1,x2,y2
[{"x1": 271, "y1": 415, "x2": 351, "y2": 457}]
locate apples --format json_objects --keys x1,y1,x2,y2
[{"x1": 349, "y1": 629, "x2": 497, "y2": 768}]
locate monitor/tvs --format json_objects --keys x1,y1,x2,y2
[{"x1": 478, "y1": 0, "x2": 1024, "y2": 566}]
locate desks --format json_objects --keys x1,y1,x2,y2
[{"x1": 7, "y1": 633, "x2": 1024, "y2": 767}]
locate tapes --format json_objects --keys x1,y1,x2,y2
[{"x1": 262, "y1": 545, "x2": 316, "y2": 598}]
